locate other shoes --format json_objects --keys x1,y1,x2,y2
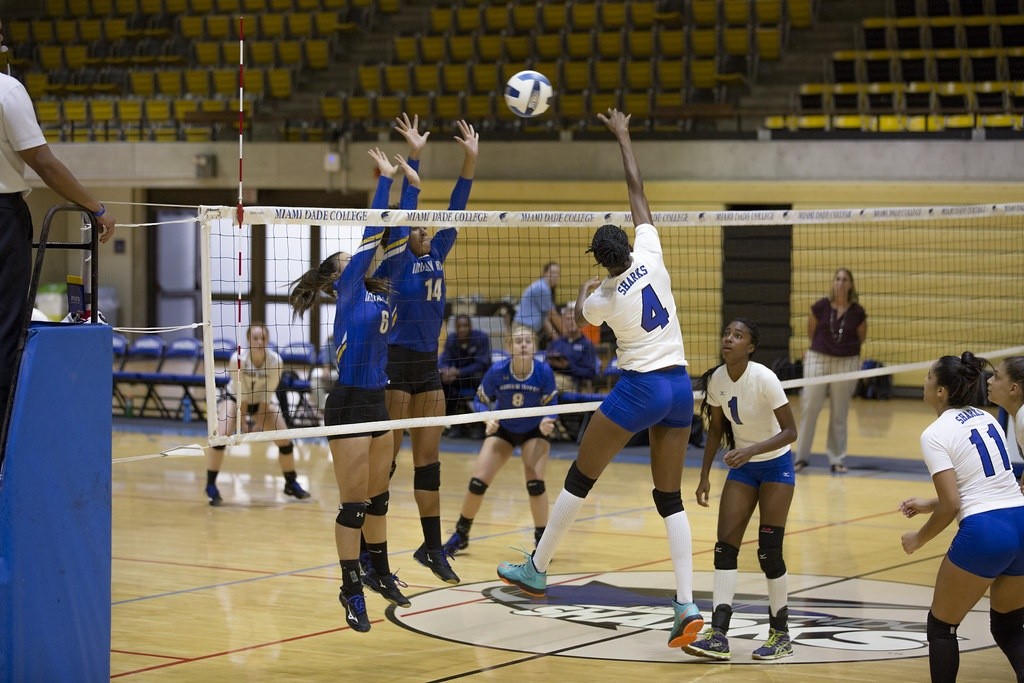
[
  {"x1": 831, "y1": 464, "x2": 848, "y2": 473},
  {"x1": 284, "y1": 484, "x2": 310, "y2": 498},
  {"x1": 794, "y1": 459, "x2": 808, "y2": 472},
  {"x1": 205, "y1": 487, "x2": 222, "y2": 505}
]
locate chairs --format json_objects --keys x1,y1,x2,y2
[
  {"x1": 0, "y1": 0, "x2": 1024, "y2": 142},
  {"x1": 112, "y1": 334, "x2": 166, "y2": 418},
  {"x1": 137, "y1": 336, "x2": 202, "y2": 419},
  {"x1": 100, "y1": 335, "x2": 129, "y2": 420},
  {"x1": 173, "y1": 339, "x2": 239, "y2": 422},
  {"x1": 448, "y1": 351, "x2": 619, "y2": 447},
  {"x1": 272, "y1": 341, "x2": 318, "y2": 425}
]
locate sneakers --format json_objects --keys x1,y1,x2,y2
[
  {"x1": 339, "y1": 586, "x2": 372, "y2": 632},
  {"x1": 360, "y1": 568, "x2": 411, "y2": 608},
  {"x1": 668, "y1": 594, "x2": 704, "y2": 647},
  {"x1": 496, "y1": 545, "x2": 547, "y2": 597},
  {"x1": 681, "y1": 628, "x2": 731, "y2": 660},
  {"x1": 413, "y1": 542, "x2": 460, "y2": 584},
  {"x1": 443, "y1": 531, "x2": 469, "y2": 554},
  {"x1": 752, "y1": 628, "x2": 793, "y2": 659}
]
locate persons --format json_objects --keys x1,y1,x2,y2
[
  {"x1": 497, "y1": 108, "x2": 705, "y2": 647},
  {"x1": 899, "y1": 352, "x2": 1024, "y2": 683},
  {"x1": 287, "y1": 110, "x2": 620, "y2": 632},
  {"x1": 0, "y1": 72, "x2": 115, "y2": 428},
  {"x1": 682, "y1": 317, "x2": 798, "y2": 660},
  {"x1": 206, "y1": 324, "x2": 310, "y2": 506},
  {"x1": 792, "y1": 268, "x2": 869, "y2": 474}
]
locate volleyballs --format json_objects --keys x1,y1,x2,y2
[{"x1": 504, "y1": 69, "x2": 555, "y2": 117}]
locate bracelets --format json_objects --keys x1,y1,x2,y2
[{"x1": 92, "y1": 202, "x2": 105, "y2": 216}]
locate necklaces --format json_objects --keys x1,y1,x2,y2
[{"x1": 831, "y1": 300, "x2": 844, "y2": 343}]
